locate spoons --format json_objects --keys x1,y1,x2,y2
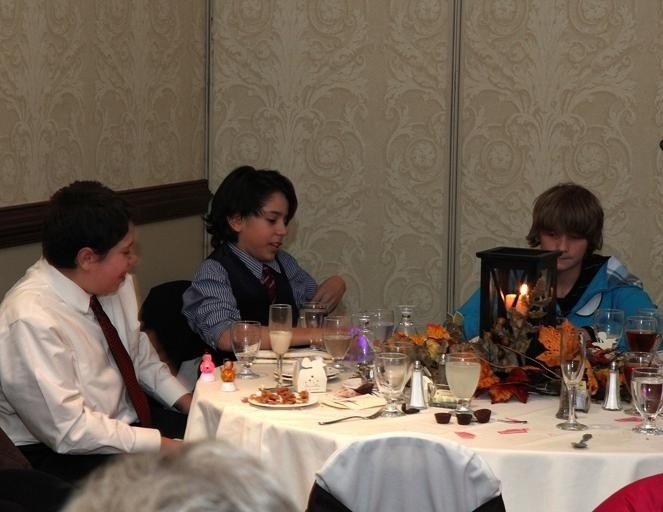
[{"x1": 571, "y1": 434, "x2": 592, "y2": 449}]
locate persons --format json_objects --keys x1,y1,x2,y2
[
  {"x1": 443, "y1": 180, "x2": 662, "y2": 357},
  {"x1": 180, "y1": 164, "x2": 362, "y2": 354},
  {"x1": 0, "y1": 180, "x2": 193, "y2": 481}
]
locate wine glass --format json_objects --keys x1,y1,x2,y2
[
  {"x1": 445, "y1": 353, "x2": 482, "y2": 418},
  {"x1": 268, "y1": 300, "x2": 418, "y2": 390},
  {"x1": 592, "y1": 307, "x2": 663, "y2": 436},
  {"x1": 232, "y1": 320, "x2": 260, "y2": 382},
  {"x1": 556, "y1": 329, "x2": 587, "y2": 433},
  {"x1": 373, "y1": 352, "x2": 412, "y2": 417}
]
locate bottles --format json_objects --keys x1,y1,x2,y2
[
  {"x1": 437, "y1": 354, "x2": 447, "y2": 389},
  {"x1": 409, "y1": 360, "x2": 427, "y2": 411},
  {"x1": 555, "y1": 363, "x2": 578, "y2": 421}
]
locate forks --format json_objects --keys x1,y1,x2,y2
[{"x1": 317, "y1": 407, "x2": 385, "y2": 425}]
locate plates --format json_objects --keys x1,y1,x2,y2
[{"x1": 247, "y1": 393, "x2": 315, "y2": 410}]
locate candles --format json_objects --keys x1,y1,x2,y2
[{"x1": 504, "y1": 282, "x2": 530, "y2": 315}]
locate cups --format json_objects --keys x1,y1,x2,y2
[
  {"x1": 456, "y1": 414, "x2": 471, "y2": 424},
  {"x1": 434, "y1": 411, "x2": 451, "y2": 425},
  {"x1": 473, "y1": 409, "x2": 490, "y2": 422}
]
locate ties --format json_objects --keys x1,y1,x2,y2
[
  {"x1": 261, "y1": 263, "x2": 279, "y2": 306},
  {"x1": 88, "y1": 294, "x2": 155, "y2": 430}
]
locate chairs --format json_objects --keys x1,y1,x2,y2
[
  {"x1": 306, "y1": 432, "x2": 505, "y2": 512},
  {"x1": 140, "y1": 280, "x2": 195, "y2": 439},
  {"x1": 591, "y1": 474, "x2": 661, "y2": 510}
]
